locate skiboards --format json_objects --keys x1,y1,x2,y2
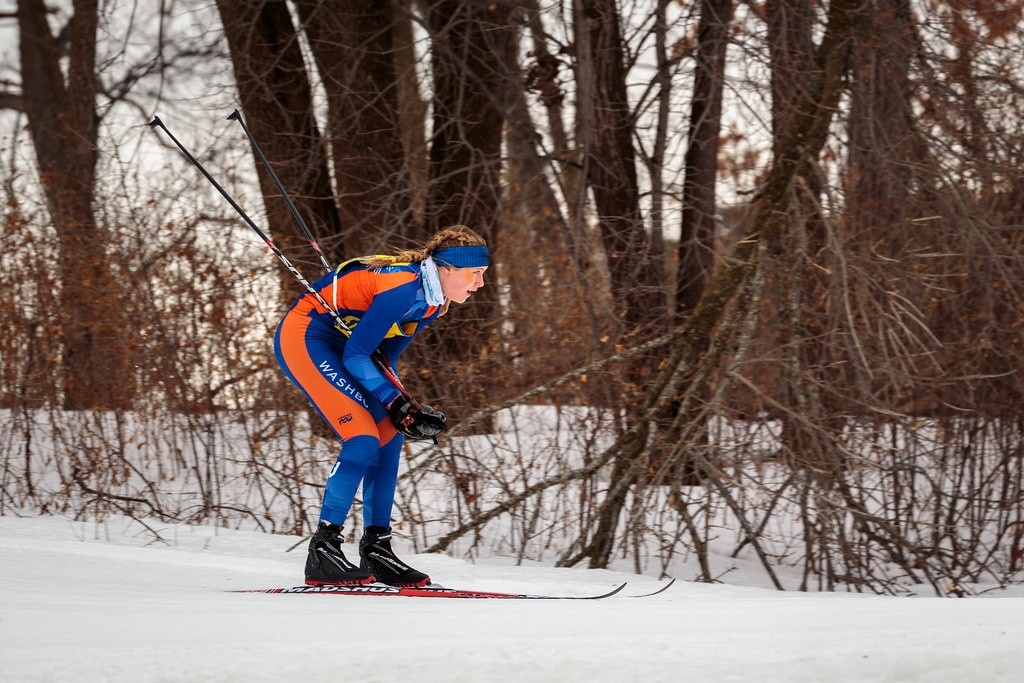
[{"x1": 223, "y1": 575, "x2": 679, "y2": 603}]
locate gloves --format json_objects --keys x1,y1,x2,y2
[{"x1": 390, "y1": 396, "x2": 446, "y2": 439}]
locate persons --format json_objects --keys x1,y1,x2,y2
[{"x1": 274, "y1": 225, "x2": 488, "y2": 588}]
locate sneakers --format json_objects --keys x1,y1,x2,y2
[
  {"x1": 359, "y1": 525, "x2": 431, "y2": 588},
  {"x1": 305, "y1": 521, "x2": 374, "y2": 587}
]
